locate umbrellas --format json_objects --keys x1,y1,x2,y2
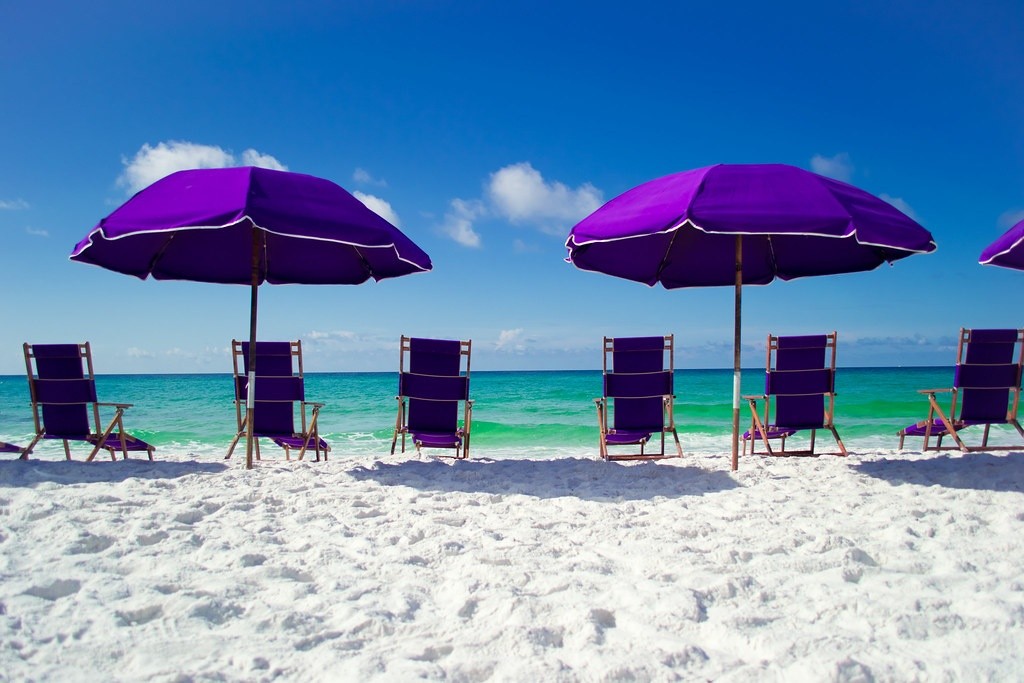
[
  {"x1": 563, "y1": 162, "x2": 937, "y2": 472},
  {"x1": 69, "y1": 165, "x2": 433, "y2": 469},
  {"x1": 978, "y1": 218, "x2": 1024, "y2": 272}
]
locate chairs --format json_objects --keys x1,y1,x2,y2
[
  {"x1": 225, "y1": 339, "x2": 332, "y2": 462},
  {"x1": 740, "y1": 331, "x2": 849, "y2": 458},
  {"x1": 391, "y1": 335, "x2": 476, "y2": 460},
  {"x1": 592, "y1": 333, "x2": 685, "y2": 462},
  {"x1": 895, "y1": 327, "x2": 1024, "y2": 453},
  {"x1": 18, "y1": 341, "x2": 156, "y2": 462}
]
